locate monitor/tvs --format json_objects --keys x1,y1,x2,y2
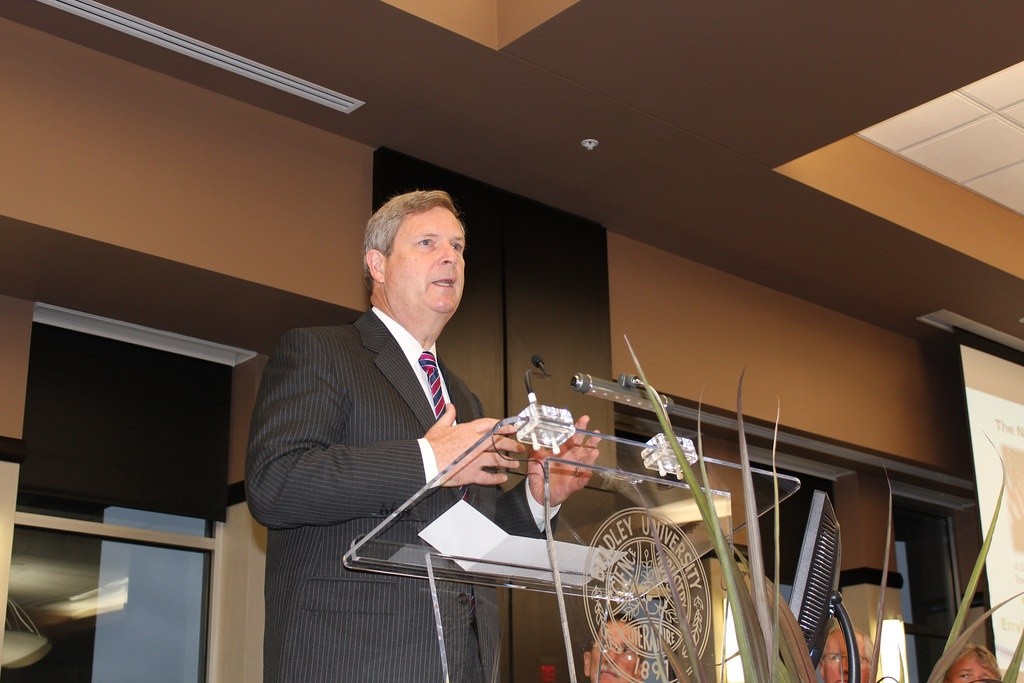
[{"x1": 786, "y1": 490, "x2": 861, "y2": 683}]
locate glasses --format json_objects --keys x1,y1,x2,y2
[
  {"x1": 591, "y1": 640, "x2": 639, "y2": 662},
  {"x1": 821, "y1": 653, "x2": 870, "y2": 665}
]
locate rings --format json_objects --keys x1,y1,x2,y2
[{"x1": 575, "y1": 467, "x2": 581, "y2": 477}]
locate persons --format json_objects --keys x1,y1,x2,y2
[
  {"x1": 944, "y1": 641, "x2": 1002, "y2": 683},
  {"x1": 817, "y1": 623, "x2": 874, "y2": 683},
  {"x1": 243, "y1": 188, "x2": 601, "y2": 683},
  {"x1": 582, "y1": 613, "x2": 646, "y2": 683}
]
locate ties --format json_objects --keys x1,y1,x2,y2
[{"x1": 418, "y1": 351, "x2": 467, "y2": 504}]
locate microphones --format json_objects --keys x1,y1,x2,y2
[{"x1": 525, "y1": 354, "x2": 552, "y2": 404}]
